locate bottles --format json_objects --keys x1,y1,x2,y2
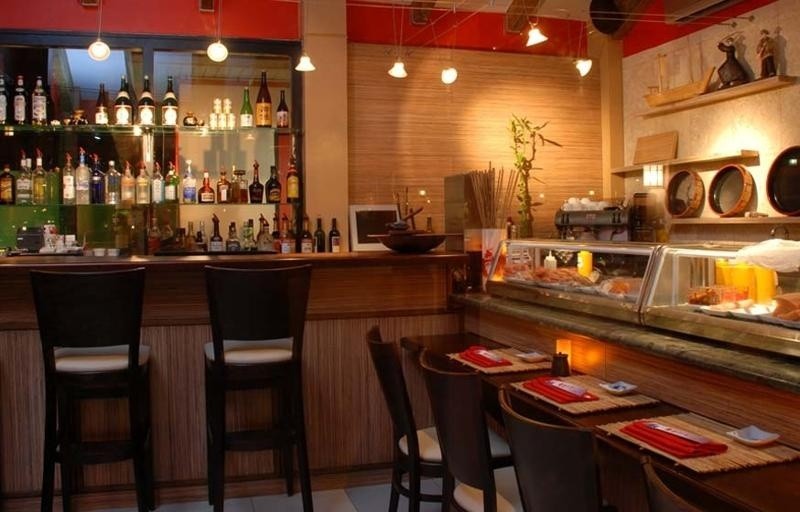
[
  {"x1": 425, "y1": 216, "x2": 435, "y2": 234},
  {"x1": 0, "y1": 69, "x2": 292, "y2": 129},
  {"x1": 551, "y1": 352, "x2": 570, "y2": 377},
  {"x1": 545, "y1": 250, "x2": 594, "y2": 280},
  {"x1": 146, "y1": 214, "x2": 341, "y2": 252},
  {"x1": 713, "y1": 258, "x2": 778, "y2": 306},
  {"x1": 505, "y1": 215, "x2": 519, "y2": 240}
]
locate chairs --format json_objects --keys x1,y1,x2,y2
[
  {"x1": 406, "y1": 322, "x2": 706, "y2": 512},
  {"x1": 370, "y1": 326, "x2": 513, "y2": 511},
  {"x1": 26, "y1": 267, "x2": 158, "y2": 512},
  {"x1": 201, "y1": 264, "x2": 317, "y2": 512}
]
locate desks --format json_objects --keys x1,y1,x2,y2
[{"x1": 399, "y1": 330, "x2": 800, "y2": 512}]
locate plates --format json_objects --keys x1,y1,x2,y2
[
  {"x1": 726, "y1": 423, "x2": 781, "y2": 449},
  {"x1": 517, "y1": 351, "x2": 548, "y2": 363},
  {"x1": 599, "y1": 380, "x2": 637, "y2": 395}
]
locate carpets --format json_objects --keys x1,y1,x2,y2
[{"x1": 93, "y1": 477, "x2": 455, "y2": 512}]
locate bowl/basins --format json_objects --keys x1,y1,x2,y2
[
  {"x1": 664, "y1": 144, "x2": 799, "y2": 218},
  {"x1": 377, "y1": 234, "x2": 445, "y2": 252}
]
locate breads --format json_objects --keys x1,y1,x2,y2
[{"x1": 711, "y1": 299, "x2": 755, "y2": 311}]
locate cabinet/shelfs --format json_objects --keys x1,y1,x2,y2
[
  {"x1": 611, "y1": 74, "x2": 800, "y2": 226},
  {"x1": 488, "y1": 243, "x2": 799, "y2": 359},
  {"x1": 1, "y1": 122, "x2": 299, "y2": 255}
]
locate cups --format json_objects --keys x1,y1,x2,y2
[
  {"x1": 92, "y1": 247, "x2": 120, "y2": 256},
  {"x1": 42, "y1": 224, "x2": 76, "y2": 249},
  {"x1": 712, "y1": 284, "x2": 751, "y2": 310}
]
